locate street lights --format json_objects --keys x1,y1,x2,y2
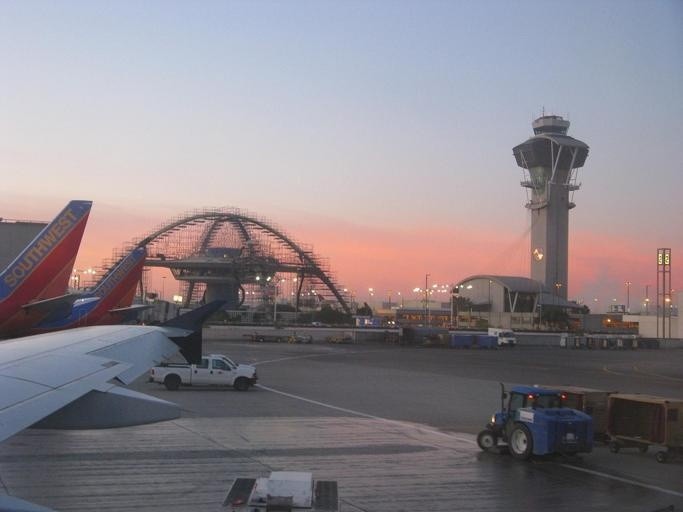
[
  {"x1": 555, "y1": 281, "x2": 563, "y2": 304},
  {"x1": 645, "y1": 285, "x2": 653, "y2": 316},
  {"x1": 367, "y1": 282, "x2": 474, "y2": 330},
  {"x1": 425, "y1": 274, "x2": 430, "y2": 318},
  {"x1": 162, "y1": 276, "x2": 166, "y2": 300},
  {"x1": 72, "y1": 274, "x2": 78, "y2": 292},
  {"x1": 623, "y1": 279, "x2": 633, "y2": 314}
]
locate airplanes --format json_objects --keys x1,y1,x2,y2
[{"x1": 1, "y1": 190, "x2": 228, "y2": 512}]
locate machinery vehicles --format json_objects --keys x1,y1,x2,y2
[{"x1": 473, "y1": 378, "x2": 597, "y2": 460}]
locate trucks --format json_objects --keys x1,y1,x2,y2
[
  {"x1": 146, "y1": 354, "x2": 258, "y2": 392},
  {"x1": 486, "y1": 326, "x2": 520, "y2": 346}
]
